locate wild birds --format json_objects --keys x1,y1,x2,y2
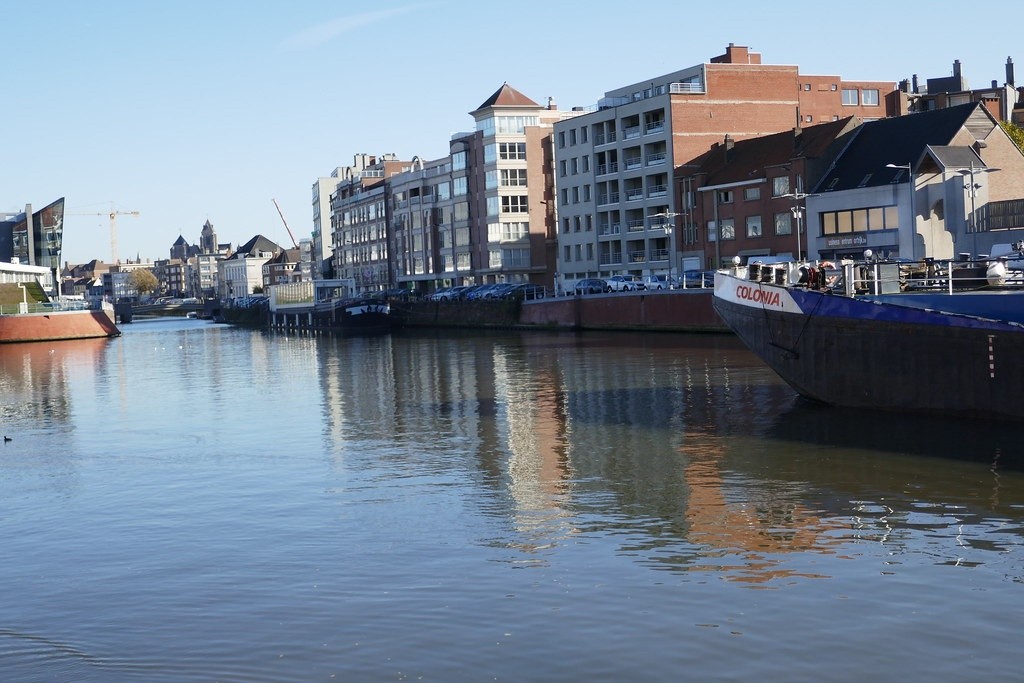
[{"x1": 3, "y1": 436, "x2": 12, "y2": 442}]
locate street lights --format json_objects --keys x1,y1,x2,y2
[
  {"x1": 963, "y1": 182, "x2": 983, "y2": 262},
  {"x1": 772, "y1": 188, "x2": 821, "y2": 261},
  {"x1": 647, "y1": 208, "x2": 689, "y2": 288},
  {"x1": 885, "y1": 163, "x2": 916, "y2": 261}
]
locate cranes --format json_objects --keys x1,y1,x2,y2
[{"x1": 63, "y1": 200, "x2": 142, "y2": 266}]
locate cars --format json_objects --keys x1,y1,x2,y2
[
  {"x1": 607, "y1": 275, "x2": 647, "y2": 293},
  {"x1": 644, "y1": 274, "x2": 679, "y2": 291},
  {"x1": 575, "y1": 279, "x2": 608, "y2": 296},
  {"x1": 355, "y1": 281, "x2": 549, "y2": 304},
  {"x1": 678, "y1": 269, "x2": 713, "y2": 288}
]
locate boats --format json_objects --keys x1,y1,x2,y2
[{"x1": 711, "y1": 259, "x2": 1024, "y2": 425}]
